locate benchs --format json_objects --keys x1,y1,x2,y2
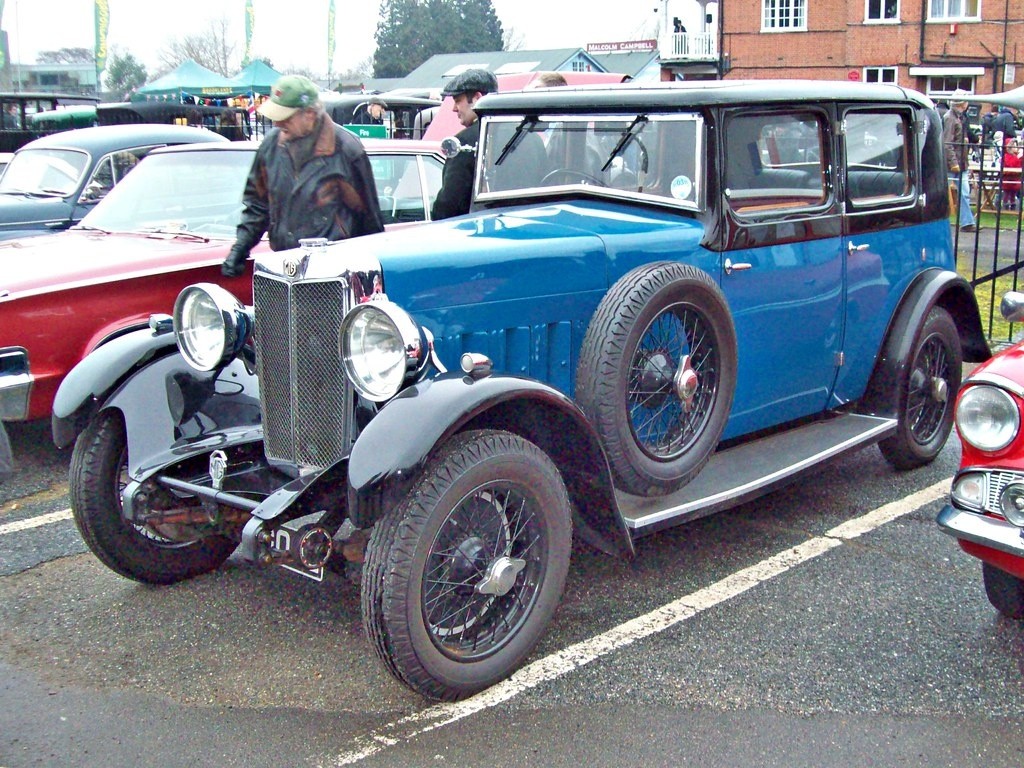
[{"x1": 750, "y1": 165, "x2": 906, "y2": 201}]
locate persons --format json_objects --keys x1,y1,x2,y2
[
  {"x1": 429, "y1": 67, "x2": 603, "y2": 226},
  {"x1": 184, "y1": 96, "x2": 247, "y2": 142},
  {"x1": 421, "y1": 122, "x2": 430, "y2": 139},
  {"x1": 394, "y1": 119, "x2": 410, "y2": 138},
  {"x1": 351, "y1": 96, "x2": 388, "y2": 125},
  {"x1": 674, "y1": 17, "x2": 689, "y2": 54},
  {"x1": 2, "y1": 104, "x2": 19, "y2": 129},
  {"x1": 219, "y1": 72, "x2": 386, "y2": 275},
  {"x1": 944, "y1": 88, "x2": 1024, "y2": 232}
]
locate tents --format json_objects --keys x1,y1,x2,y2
[
  {"x1": 227, "y1": 60, "x2": 287, "y2": 97},
  {"x1": 137, "y1": 57, "x2": 251, "y2": 104}
]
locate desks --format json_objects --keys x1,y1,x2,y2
[{"x1": 971, "y1": 167, "x2": 1022, "y2": 216}]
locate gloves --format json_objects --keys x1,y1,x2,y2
[
  {"x1": 222, "y1": 245, "x2": 249, "y2": 278},
  {"x1": 951, "y1": 165, "x2": 959, "y2": 172}
]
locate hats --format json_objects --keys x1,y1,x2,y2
[
  {"x1": 257, "y1": 75, "x2": 318, "y2": 121},
  {"x1": 439, "y1": 69, "x2": 499, "y2": 97},
  {"x1": 369, "y1": 97, "x2": 388, "y2": 108}
]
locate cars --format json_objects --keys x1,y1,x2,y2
[
  {"x1": 52, "y1": 77, "x2": 993, "y2": 705},
  {"x1": 0, "y1": 70, "x2": 791, "y2": 424},
  {"x1": 937, "y1": 289, "x2": 1024, "y2": 623}
]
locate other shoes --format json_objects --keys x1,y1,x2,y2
[{"x1": 960, "y1": 225, "x2": 982, "y2": 231}]
